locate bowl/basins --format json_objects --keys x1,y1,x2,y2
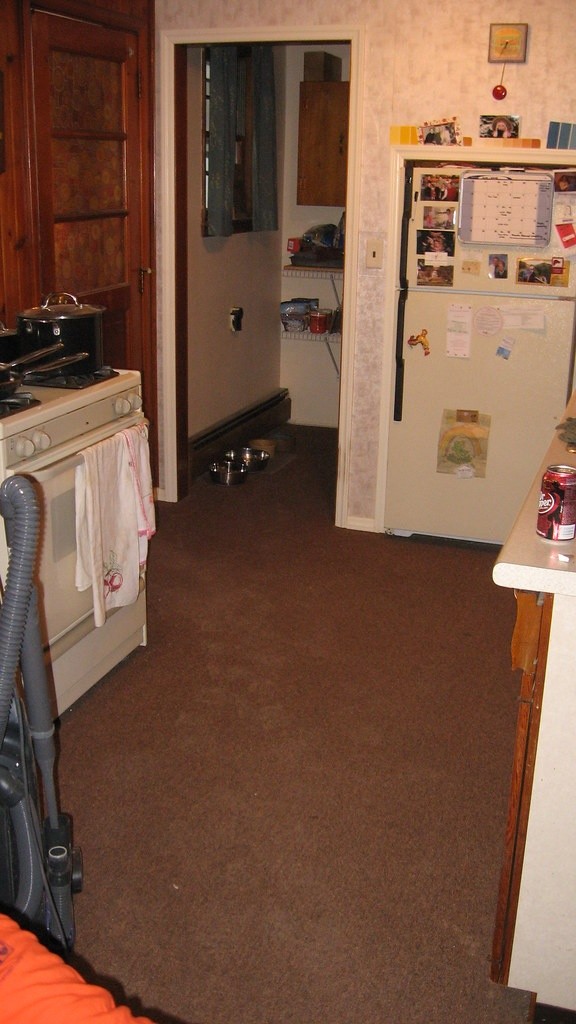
[{"x1": 208, "y1": 439, "x2": 277, "y2": 484}]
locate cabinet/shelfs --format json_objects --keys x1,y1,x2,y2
[
  {"x1": 490, "y1": 386, "x2": 576, "y2": 1024},
  {"x1": 274, "y1": 81, "x2": 350, "y2": 343}
]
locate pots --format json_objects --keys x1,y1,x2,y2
[
  {"x1": 17, "y1": 292, "x2": 107, "y2": 377},
  {"x1": 0, "y1": 343, "x2": 88, "y2": 399}
]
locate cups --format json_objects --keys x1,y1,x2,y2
[{"x1": 311, "y1": 309, "x2": 333, "y2": 333}]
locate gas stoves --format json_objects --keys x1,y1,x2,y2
[{"x1": 0, "y1": 364, "x2": 143, "y2": 468}]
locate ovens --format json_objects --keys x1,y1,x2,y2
[{"x1": 0, "y1": 412, "x2": 146, "y2": 722}]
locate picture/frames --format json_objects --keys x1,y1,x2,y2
[{"x1": 416, "y1": 115, "x2": 461, "y2": 145}]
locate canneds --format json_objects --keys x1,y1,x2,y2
[{"x1": 536, "y1": 465, "x2": 576, "y2": 542}]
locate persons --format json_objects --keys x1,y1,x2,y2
[
  {"x1": 492, "y1": 120, "x2": 511, "y2": 138},
  {"x1": 424, "y1": 126, "x2": 451, "y2": 146},
  {"x1": 488, "y1": 257, "x2": 507, "y2": 279},
  {"x1": 559, "y1": 175, "x2": 574, "y2": 191},
  {"x1": 424, "y1": 209, "x2": 454, "y2": 229},
  {"x1": 424, "y1": 182, "x2": 456, "y2": 201}
]
